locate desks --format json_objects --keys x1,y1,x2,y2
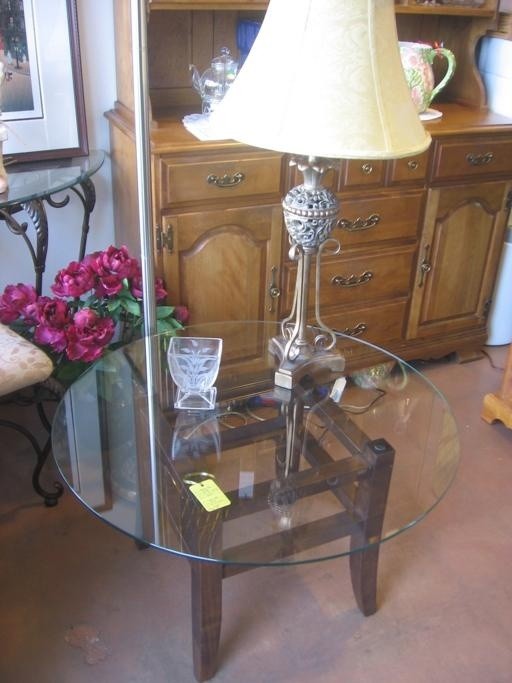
[
  {"x1": 52, "y1": 320, "x2": 461, "y2": 682},
  {"x1": 1, "y1": 150, "x2": 106, "y2": 300}
]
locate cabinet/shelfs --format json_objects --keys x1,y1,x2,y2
[{"x1": 103, "y1": 0, "x2": 512, "y2": 405}]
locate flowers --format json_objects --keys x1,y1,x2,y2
[{"x1": 1, "y1": 246, "x2": 190, "y2": 401}]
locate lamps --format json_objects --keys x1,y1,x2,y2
[{"x1": 210, "y1": 0, "x2": 433, "y2": 388}]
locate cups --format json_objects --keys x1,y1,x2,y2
[{"x1": 202, "y1": 98, "x2": 220, "y2": 119}]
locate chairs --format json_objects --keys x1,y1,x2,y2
[{"x1": 0, "y1": 324, "x2": 62, "y2": 508}]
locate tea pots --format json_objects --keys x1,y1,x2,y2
[{"x1": 191, "y1": 46, "x2": 240, "y2": 103}]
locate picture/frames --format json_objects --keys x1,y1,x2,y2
[
  {"x1": 1, "y1": 1, "x2": 90, "y2": 161},
  {"x1": 52, "y1": 360, "x2": 112, "y2": 513}
]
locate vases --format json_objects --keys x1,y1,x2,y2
[{"x1": 73, "y1": 379, "x2": 147, "y2": 447}]
